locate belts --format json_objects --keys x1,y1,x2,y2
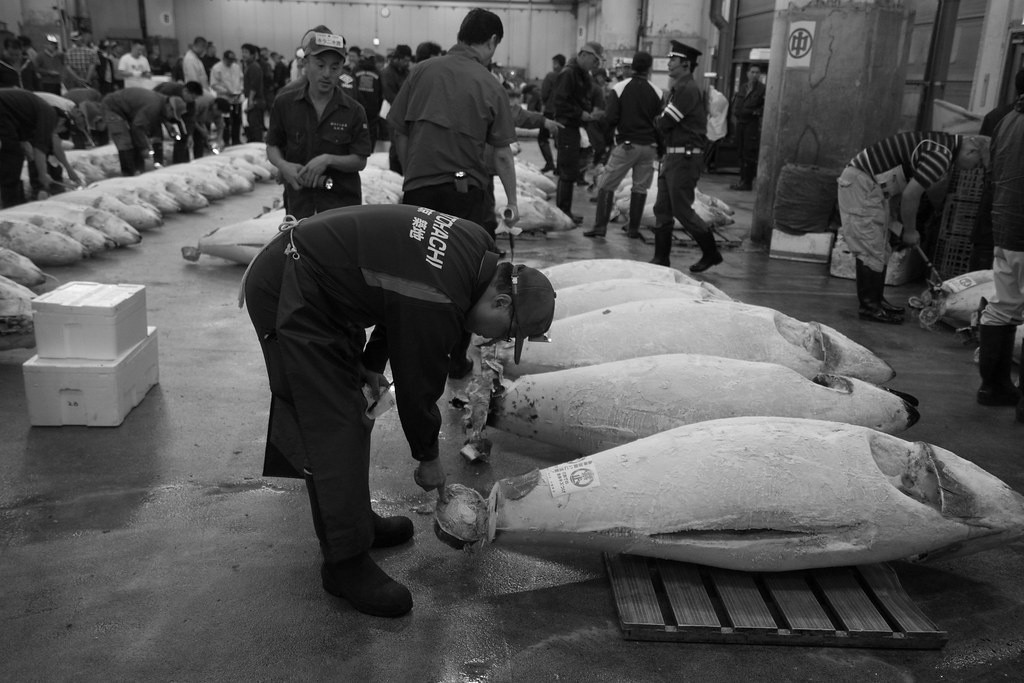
[{"x1": 666, "y1": 146, "x2": 701, "y2": 155}]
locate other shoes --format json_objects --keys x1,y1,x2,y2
[
  {"x1": 321, "y1": 558, "x2": 413, "y2": 619},
  {"x1": 728, "y1": 183, "x2": 752, "y2": 192},
  {"x1": 373, "y1": 515, "x2": 414, "y2": 550}
]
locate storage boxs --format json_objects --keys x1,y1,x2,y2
[
  {"x1": 828, "y1": 230, "x2": 917, "y2": 287},
  {"x1": 20, "y1": 326, "x2": 161, "y2": 427},
  {"x1": 30, "y1": 280, "x2": 149, "y2": 359},
  {"x1": 934, "y1": 162, "x2": 988, "y2": 282},
  {"x1": 770, "y1": 229, "x2": 836, "y2": 263}
]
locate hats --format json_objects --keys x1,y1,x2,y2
[
  {"x1": 69, "y1": 29, "x2": 82, "y2": 41},
  {"x1": 170, "y1": 95, "x2": 186, "y2": 122},
  {"x1": 360, "y1": 46, "x2": 376, "y2": 59},
  {"x1": 224, "y1": 50, "x2": 237, "y2": 62},
  {"x1": 976, "y1": 134, "x2": 992, "y2": 170},
  {"x1": 303, "y1": 32, "x2": 347, "y2": 64},
  {"x1": 582, "y1": 40, "x2": 606, "y2": 61},
  {"x1": 386, "y1": 47, "x2": 396, "y2": 56},
  {"x1": 59, "y1": 99, "x2": 99, "y2": 140},
  {"x1": 511, "y1": 263, "x2": 557, "y2": 366},
  {"x1": 664, "y1": 38, "x2": 703, "y2": 62}
]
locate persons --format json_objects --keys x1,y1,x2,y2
[
  {"x1": 727, "y1": 62, "x2": 766, "y2": 191},
  {"x1": 836, "y1": 130, "x2": 991, "y2": 325},
  {"x1": 0, "y1": 27, "x2": 665, "y2": 237},
  {"x1": 968, "y1": 68, "x2": 1024, "y2": 425},
  {"x1": 358, "y1": 7, "x2": 519, "y2": 384},
  {"x1": 266, "y1": 33, "x2": 372, "y2": 223},
  {"x1": 238, "y1": 203, "x2": 556, "y2": 619},
  {"x1": 648, "y1": 40, "x2": 723, "y2": 274}
]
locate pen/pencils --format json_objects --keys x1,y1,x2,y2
[{"x1": 366, "y1": 381, "x2": 394, "y2": 414}]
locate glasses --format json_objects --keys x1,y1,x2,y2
[{"x1": 501, "y1": 306, "x2": 516, "y2": 343}]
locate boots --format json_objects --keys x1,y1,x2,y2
[
  {"x1": 622, "y1": 191, "x2": 647, "y2": 239},
  {"x1": 856, "y1": 261, "x2": 906, "y2": 324},
  {"x1": 536, "y1": 141, "x2": 591, "y2": 223},
  {"x1": 448, "y1": 328, "x2": 473, "y2": 380},
  {"x1": 689, "y1": 226, "x2": 722, "y2": 272},
  {"x1": 977, "y1": 323, "x2": 1024, "y2": 424},
  {"x1": 647, "y1": 221, "x2": 674, "y2": 267},
  {"x1": 583, "y1": 189, "x2": 614, "y2": 237}
]
